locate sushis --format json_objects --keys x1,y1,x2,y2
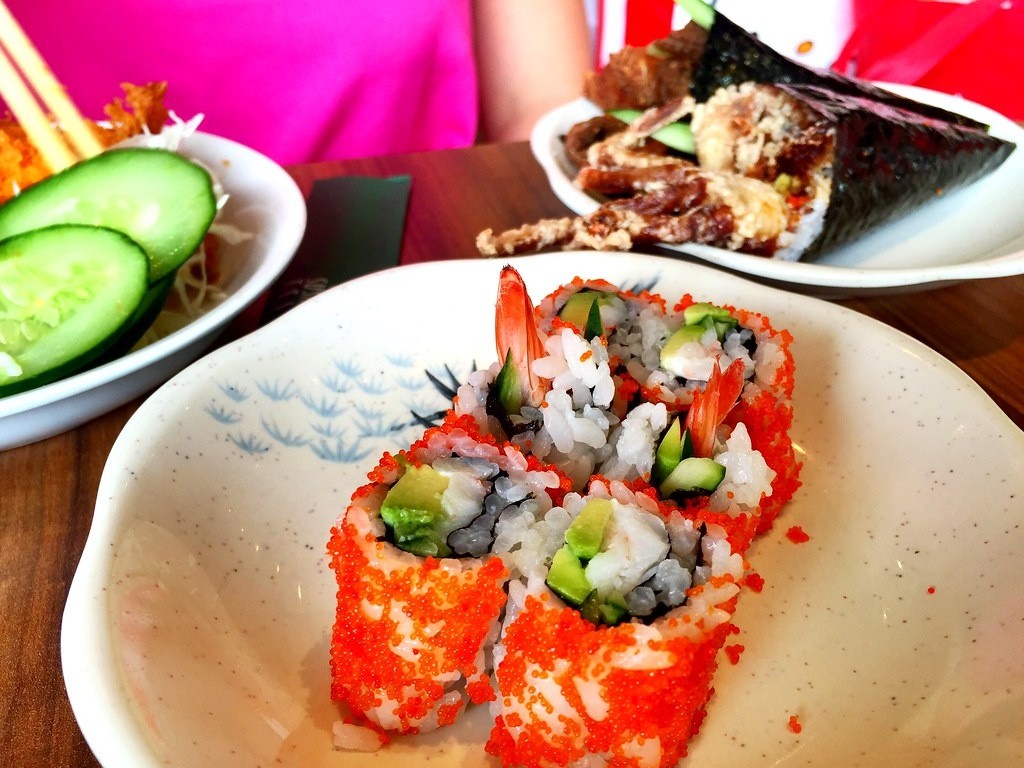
[
  {"x1": 327, "y1": 278, "x2": 803, "y2": 768},
  {"x1": 474, "y1": 0, "x2": 1015, "y2": 268}
]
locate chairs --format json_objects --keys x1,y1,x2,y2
[{"x1": 599, "y1": 0, "x2": 1024, "y2": 131}]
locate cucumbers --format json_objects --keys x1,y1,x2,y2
[{"x1": 0, "y1": 146, "x2": 215, "y2": 397}]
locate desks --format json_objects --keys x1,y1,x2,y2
[{"x1": 0, "y1": 142, "x2": 1024, "y2": 768}]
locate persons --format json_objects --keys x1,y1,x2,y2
[{"x1": 1, "y1": 0, "x2": 594, "y2": 165}]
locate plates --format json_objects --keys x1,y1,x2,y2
[
  {"x1": 529, "y1": 80, "x2": 1024, "y2": 296},
  {"x1": 62, "y1": 252, "x2": 1024, "y2": 768},
  {"x1": 0, "y1": 120, "x2": 308, "y2": 450}
]
